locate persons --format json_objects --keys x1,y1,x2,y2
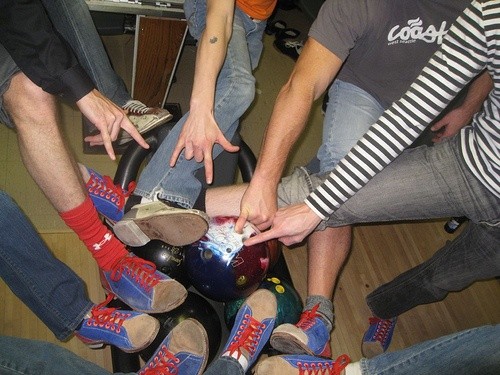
[
  {"x1": 113, "y1": 0, "x2": 277, "y2": 246},
  {"x1": 0, "y1": 0, "x2": 500, "y2": 375}
]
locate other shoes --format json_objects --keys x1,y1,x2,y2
[
  {"x1": 264, "y1": 20, "x2": 288, "y2": 35},
  {"x1": 273, "y1": 39, "x2": 304, "y2": 62},
  {"x1": 276, "y1": 28, "x2": 300, "y2": 40}
]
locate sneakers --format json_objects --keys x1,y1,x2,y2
[
  {"x1": 255, "y1": 354, "x2": 350, "y2": 375},
  {"x1": 103, "y1": 252, "x2": 188, "y2": 314},
  {"x1": 117, "y1": 100, "x2": 173, "y2": 146},
  {"x1": 361, "y1": 316, "x2": 397, "y2": 359},
  {"x1": 218, "y1": 289, "x2": 276, "y2": 374},
  {"x1": 269, "y1": 302, "x2": 332, "y2": 359},
  {"x1": 85, "y1": 169, "x2": 136, "y2": 222},
  {"x1": 136, "y1": 318, "x2": 209, "y2": 375},
  {"x1": 75, "y1": 293, "x2": 160, "y2": 353},
  {"x1": 113, "y1": 201, "x2": 209, "y2": 248}
]
